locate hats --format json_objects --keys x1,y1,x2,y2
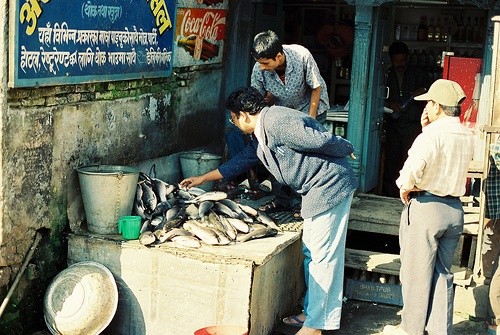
[{"x1": 414, "y1": 78, "x2": 467, "y2": 107}]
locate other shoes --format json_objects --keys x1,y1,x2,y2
[
  {"x1": 258, "y1": 179, "x2": 272, "y2": 192},
  {"x1": 238, "y1": 179, "x2": 251, "y2": 190},
  {"x1": 383, "y1": 325, "x2": 407, "y2": 335}
]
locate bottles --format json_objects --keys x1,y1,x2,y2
[
  {"x1": 328, "y1": 14, "x2": 354, "y2": 28},
  {"x1": 339, "y1": 61, "x2": 350, "y2": 80},
  {"x1": 406, "y1": 48, "x2": 483, "y2": 91},
  {"x1": 417, "y1": 16, "x2": 485, "y2": 43}
]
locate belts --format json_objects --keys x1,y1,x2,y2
[{"x1": 410, "y1": 190, "x2": 459, "y2": 199}]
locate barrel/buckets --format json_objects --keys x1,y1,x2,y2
[
  {"x1": 179, "y1": 150, "x2": 222, "y2": 192},
  {"x1": 76, "y1": 162, "x2": 140, "y2": 235},
  {"x1": 193, "y1": 324, "x2": 248, "y2": 335}
]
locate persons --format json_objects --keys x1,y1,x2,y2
[
  {"x1": 383, "y1": 79, "x2": 476, "y2": 335},
  {"x1": 221, "y1": 30, "x2": 331, "y2": 220},
  {"x1": 180, "y1": 86, "x2": 359, "y2": 335},
  {"x1": 382, "y1": 41, "x2": 431, "y2": 197}
]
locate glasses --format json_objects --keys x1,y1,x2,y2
[{"x1": 229, "y1": 112, "x2": 239, "y2": 124}]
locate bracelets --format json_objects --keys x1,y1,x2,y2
[{"x1": 423, "y1": 88, "x2": 427, "y2": 91}]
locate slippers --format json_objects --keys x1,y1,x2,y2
[
  {"x1": 257, "y1": 200, "x2": 292, "y2": 213},
  {"x1": 283, "y1": 315, "x2": 304, "y2": 327},
  {"x1": 292, "y1": 206, "x2": 303, "y2": 220}
]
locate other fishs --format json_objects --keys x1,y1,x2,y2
[{"x1": 136, "y1": 172, "x2": 280, "y2": 248}]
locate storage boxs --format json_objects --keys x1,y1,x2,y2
[{"x1": 347, "y1": 272, "x2": 403, "y2": 306}]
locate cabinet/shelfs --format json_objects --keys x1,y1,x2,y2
[{"x1": 280, "y1": 1, "x2": 496, "y2": 195}]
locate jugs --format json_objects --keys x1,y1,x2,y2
[{"x1": 117, "y1": 216, "x2": 142, "y2": 240}]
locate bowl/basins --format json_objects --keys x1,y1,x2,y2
[{"x1": 43, "y1": 261, "x2": 118, "y2": 335}]
